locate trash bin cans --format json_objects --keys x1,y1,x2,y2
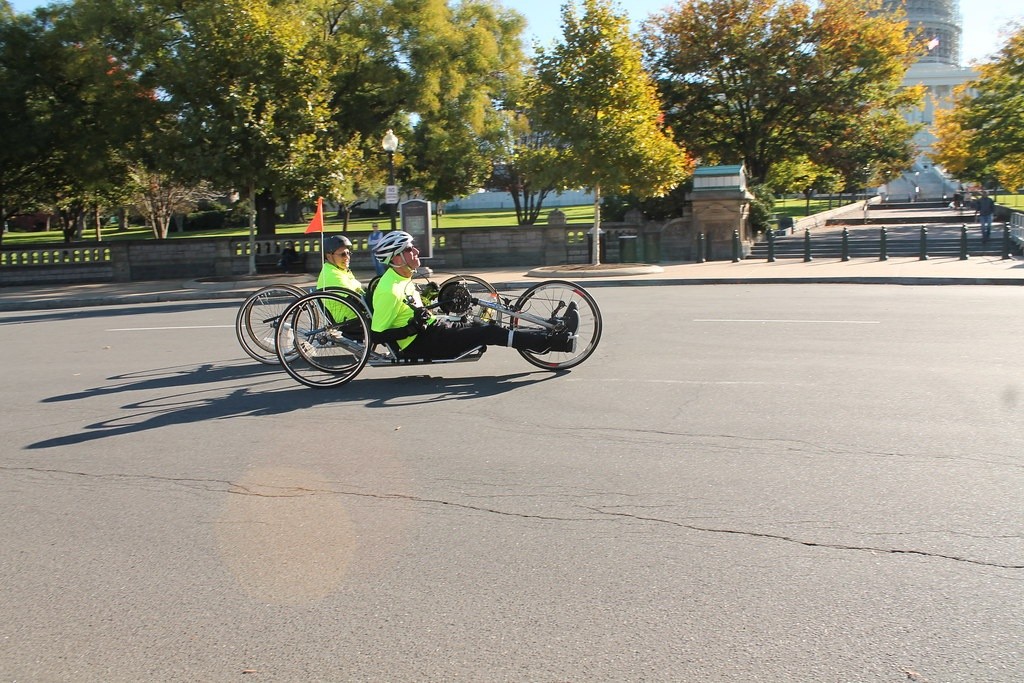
[
  {"x1": 618, "y1": 235, "x2": 637, "y2": 262},
  {"x1": 587, "y1": 228, "x2": 607, "y2": 263},
  {"x1": 642, "y1": 231, "x2": 661, "y2": 261}
]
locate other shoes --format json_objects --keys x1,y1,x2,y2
[
  {"x1": 552, "y1": 310, "x2": 580, "y2": 352},
  {"x1": 559, "y1": 300, "x2": 579, "y2": 327}
]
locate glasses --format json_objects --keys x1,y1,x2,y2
[
  {"x1": 396, "y1": 243, "x2": 415, "y2": 256},
  {"x1": 333, "y1": 251, "x2": 352, "y2": 257}
]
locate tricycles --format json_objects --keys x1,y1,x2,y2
[{"x1": 235, "y1": 271, "x2": 603, "y2": 389}]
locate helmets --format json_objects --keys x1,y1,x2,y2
[
  {"x1": 372, "y1": 231, "x2": 414, "y2": 265},
  {"x1": 323, "y1": 235, "x2": 352, "y2": 256}
]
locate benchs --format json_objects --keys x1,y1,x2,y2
[{"x1": 211, "y1": 252, "x2": 309, "y2": 275}]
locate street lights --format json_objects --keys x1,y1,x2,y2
[{"x1": 382, "y1": 127, "x2": 399, "y2": 231}]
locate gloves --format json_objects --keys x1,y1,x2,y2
[
  {"x1": 421, "y1": 281, "x2": 440, "y2": 300},
  {"x1": 405, "y1": 307, "x2": 432, "y2": 336}
]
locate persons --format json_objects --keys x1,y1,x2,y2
[
  {"x1": 950, "y1": 187, "x2": 973, "y2": 212},
  {"x1": 366, "y1": 222, "x2": 385, "y2": 277},
  {"x1": 315, "y1": 235, "x2": 495, "y2": 344},
  {"x1": 367, "y1": 230, "x2": 582, "y2": 362},
  {"x1": 975, "y1": 190, "x2": 995, "y2": 244}
]
